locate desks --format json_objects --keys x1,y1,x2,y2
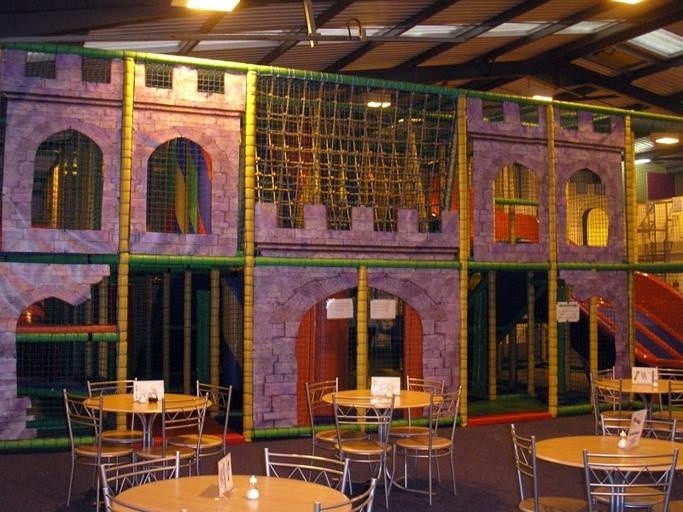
[{"x1": 114, "y1": 474, "x2": 351, "y2": 512}]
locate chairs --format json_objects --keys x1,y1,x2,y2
[
  {"x1": 263, "y1": 373, "x2": 462, "y2": 512},
  {"x1": 507, "y1": 414, "x2": 682, "y2": 512},
  {"x1": 590, "y1": 365, "x2": 683, "y2": 441},
  {"x1": 63, "y1": 375, "x2": 233, "y2": 512}
]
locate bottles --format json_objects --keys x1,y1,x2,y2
[
  {"x1": 385, "y1": 384, "x2": 394, "y2": 399},
  {"x1": 149, "y1": 389, "x2": 158, "y2": 402},
  {"x1": 139, "y1": 392, "x2": 146, "y2": 403},
  {"x1": 652, "y1": 377, "x2": 658, "y2": 387},
  {"x1": 246, "y1": 475, "x2": 259, "y2": 499},
  {"x1": 617, "y1": 430, "x2": 626, "y2": 449},
  {"x1": 369, "y1": 394, "x2": 377, "y2": 404}
]
